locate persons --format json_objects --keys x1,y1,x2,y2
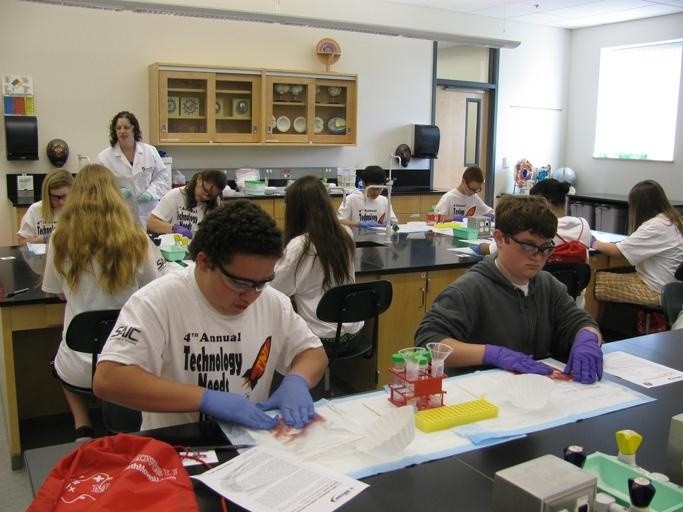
[
  {"x1": 266, "y1": 173, "x2": 364, "y2": 355},
  {"x1": 587, "y1": 179, "x2": 683, "y2": 331},
  {"x1": 17, "y1": 170, "x2": 76, "y2": 245},
  {"x1": 434, "y1": 167, "x2": 499, "y2": 225},
  {"x1": 415, "y1": 194, "x2": 604, "y2": 385},
  {"x1": 93, "y1": 200, "x2": 330, "y2": 433},
  {"x1": 150, "y1": 170, "x2": 228, "y2": 236},
  {"x1": 478, "y1": 180, "x2": 592, "y2": 318},
  {"x1": 334, "y1": 166, "x2": 398, "y2": 228},
  {"x1": 42, "y1": 162, "x2": 164, "y2": 443},
  {"x1": 99, "y1": 111, "x2": 170, "y2": 233}
]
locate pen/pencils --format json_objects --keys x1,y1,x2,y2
[
  {"x1": 188, "y1": 444, "x2": 253, "y2": 451},
  {"x1": 7, "y1": 288, "x2": 30, "y2": 297}
]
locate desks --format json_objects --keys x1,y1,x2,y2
[
  {"x1": 24, "y1": 395, "x2": 493, "y2": 511},
  {"x1": 351, "y1": 328, "x2": 682, "y2": 511},
  {"x1": 0, "y1": 257, "x2": 381, "y2": 471},
  {"x1": 354, "y1": 244, "x2": 617, "y2": 388},
  {"x1": 569, "y1": 194, "x2": 683, "y2": 234}
]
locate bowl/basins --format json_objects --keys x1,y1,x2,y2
[{"x1": 501, "y1": 373, "x2": 556, "y2": 409}]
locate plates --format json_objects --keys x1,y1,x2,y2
[{"x1": 272, "y1": 114, "x2": 347, "y2": 134}]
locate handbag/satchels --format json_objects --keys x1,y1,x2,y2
[
  {"x1": 25, "y1": 433, "x2": 230, "y2": 512},
  {"x1": 553, "y1": 229, "x2": 587, "y2": 265}
]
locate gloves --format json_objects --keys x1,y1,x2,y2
[
  {"x1": 483, "y1": 343, "x2": 554, "y2": 375},
  {"x1": 137, "y1": 191, "x2": 152, "y2": 203},
  {"x1": 200, "y1": 389, "x2": 279, "y2": 430},
  {"x1": 358, "y1": 220, "x2": 382, "y2": 228},
  {"x1": 483, "y1": 213, "x2": 494, "y2": 221},
  {"x1": 119, "y1": 187, "x2": 133, "y2": 199},
  {"x1": 563, "y1": 329, "x2": 603, "y2": 384},
  {"x1": 171, "y1": 223, "x2": 192, "y2": 239},
  {"x1": 453, "y1": 214, "x2": 466, "y2": 221},
  {"x1": 256, "y1": 374, "x2": 315, "y2": 429}
]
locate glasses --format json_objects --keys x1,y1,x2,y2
[
  {"x1": 510, "y1": 234, "x2": 557, "y2": 258},
  {"x1": 466, "y1": 183, "x2": 482, "y2": 193},
  {"x1": 50, "y1": 193, "x2": 67, "y2": 200},
  {"x1": 216, "y1": 263, "x2": 275, "y2": 293}
]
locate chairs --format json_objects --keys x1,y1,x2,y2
[
  {"x1": 660, "y1": 280, "x2": 682, "y2": 330},
  {"x1": 60, "y1": 306, "x2": 121, "y2": 402},
  {"x1": 614, "y1": 262, "x2": 682, "y2": 334},
  {"x1": 309, "y1": 280, "x2": 393, "y2": 402},
  {"x1": 542, "y1": 262, "x2": 592, "y2": 302}
]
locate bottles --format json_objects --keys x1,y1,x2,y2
[
  {"x1": 357, "y1": 179, "x2": 363, "y2": 191},
  {"x1": 437, "y1": 209, "x2": 447, "y2": 224},
  {"x1": 564, "y1": 429, "x2": 677, "y2": 512},
  {"x1": 174, "y1": 235, "x2": 190, "y2": 255},
  {"x1": 427, "y1": 210, "x2": 434, "y2": 226},
  {"x1": 391, "y1": 343, "x2": 454, "y2": 407}
]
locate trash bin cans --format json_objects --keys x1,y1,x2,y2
[{"x1": 569, "y1": 200, "x2": 629, "y2": 234}]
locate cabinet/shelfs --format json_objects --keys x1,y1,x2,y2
[
  {"x1": 264, "y1": 68, "x2": 359, "y2": 147},
  {"x1": 148, "y1": 62, "x2": 264, "y2": 147},
  {"x1": 13, "y1": 195, "x2": 275, "y2": 244},
  {"x1": 275, "y1": 192, "x2": 447, "y2": 231}
]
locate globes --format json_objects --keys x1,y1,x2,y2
[{"x1": 553, "y1": 167, "x2": 576, "y2": 186}]
